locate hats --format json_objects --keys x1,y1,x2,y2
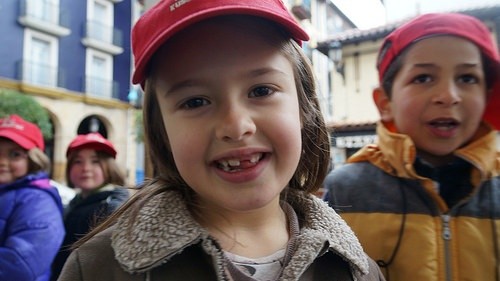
[
  {"x1": 1, "y1": 114, "x2": 45, "y2": 153},
  {"x1": 66, "y1": 132, "x2": 118, "y2": 158},
  {"x1": 376, "y1": 13, "x2": 500, "y2": 132},
  {"x1": 132, "y1": 0, "x2": 310, "y2": 94}
]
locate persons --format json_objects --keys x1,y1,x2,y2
[
  {"x1": 1, "y1": 108, "x2": 65, "y2": 281},
  {"x1": 56, "y1": 133, "x2": 134, "y2": 281},
  {"x1": 321, "y1": 11, "x2": 500, "y2": 281},
  {"x1": 52, "y1": 0, "x2": 384, "y2": 280}
]
